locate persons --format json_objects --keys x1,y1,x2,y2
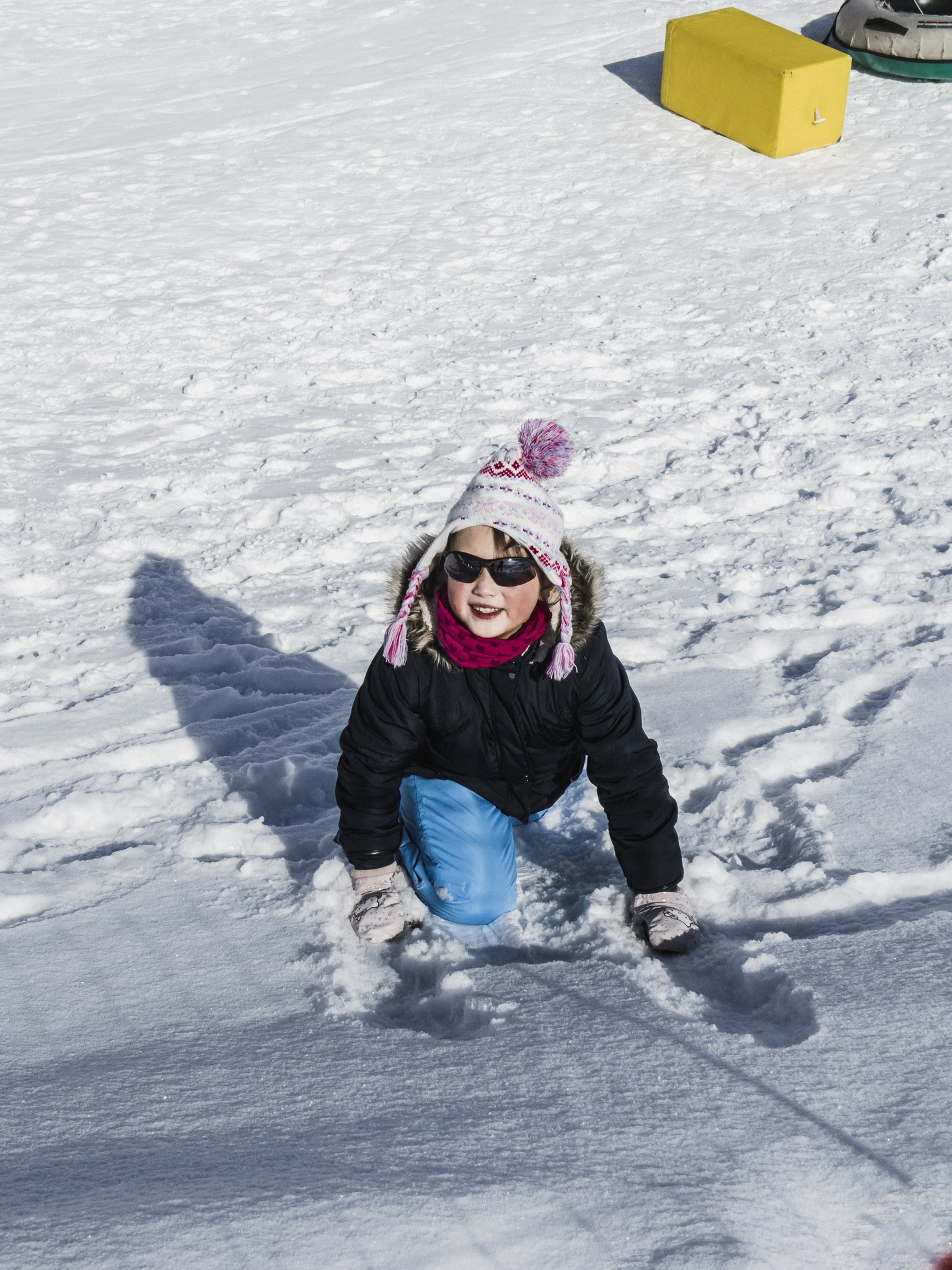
[{"x1": 337, "y1": 416, "x2": 706, "y2": 953}]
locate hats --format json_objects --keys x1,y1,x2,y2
[{"x1": 382, "y1": 419, "x2": 577, "y2": 681}]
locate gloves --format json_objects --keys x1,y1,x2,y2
[
  {"x1": 630, "y1": 886, "x2": 699, "y2": 953},
  {"x1": 350, "y1": 873, "x2": 422, "y2": 942}
]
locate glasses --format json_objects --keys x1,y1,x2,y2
[{"x1": 443, "y1": 550, "x2": 538, "y2": 588}]
